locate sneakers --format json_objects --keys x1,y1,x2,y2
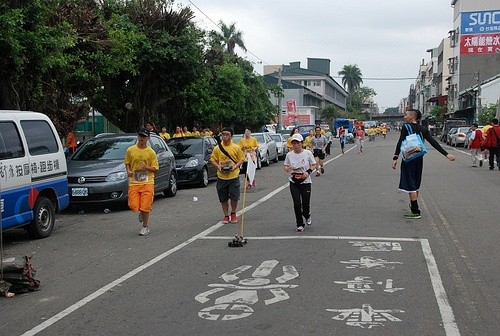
[
  {"x1": 409, "y1": 204, "x2": 413, "y2": 213},
  {"x1": 139, "y1": 212, "x2": 143, "y2": 222},
  {"x1": 139, "y1": 226, "x2": 150, "y2": 236},
  {"x1": 223, "y1": 217, "x2": 229, "y2": 224},
  {"x1": 306, "y1": 215, "x2": 312, "y2": 226},
  {"x1": 404, "y1": 211, "x2": 421, "y2": 219},
  {"x1": 296, "y1": 226, "x2": 304, "y2": 232},
  {"x1": 231, "y1": 214, "x2": 237, "y2": 222}
]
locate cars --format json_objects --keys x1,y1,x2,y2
[
  {"x1": 266, "y1": 123, "x2": 323, "y2": 155},
  {"x1": 165, "y1": 136, "x2": 221, "y2": 187},
  {"x1": 66, "y1": 132, "x2": 178, "y2": 202},
  {"x1": 426, "y1": 119, "x2": 485, "y2": 148},
  {"x1": 226, "y1": 134, "x2": 262, "y2": 173},
  {"x1": 249, "y1": 132, "x2": 279, "y2": 166},
  {"x1": 269, "y1": 134, "x2": 286, "y2": 161}
]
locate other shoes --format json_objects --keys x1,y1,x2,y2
[
  {"x1": 316, "y1": 172, "x2": 320, "y2": 176},
  {"x1": 252, "y1": 181, "x2": 255, "y2": 187},
  {"x1": 489, "y1": 166, "x2": 494, "y2": 170},
  {"x1": 246, "y1": 184, "x2": 251, "y2": 189},
  {"x1": 472, "y1": 165, "x2": 477, "y2": 167},
  {"x1": 480, "y1": 160, "x2": 482, "y2": 166},
  {"x1": 321, "y1": 168, "x2": 324, "y2": 173}
]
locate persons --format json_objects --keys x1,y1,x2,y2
[
  {"x1": 286, "y1": 126, "x2": 333, "y2": 176},
  {"x1": 284, "y1": 133, "x2": 316, "y2": 232},
  {"x1": 392, "y1": 109, "x2": 456, "y2": 219},
  {"x1": 466, "y1": 118, "x2": 500, "y2": 171},
  {"x1": 65, "y1": 128, "x2": 77, "y2": 156},
  {"x1": 237, "y1": 128, "x2": 259, "y2": 190},
  {"x1": 125, "y1": 127, "x2": 159, "y2": 235},
  {"x1": 338, "y1": 124, "x2": 388, "y2": 154},
  {"x1": 147, "y1": 122, "x2": 221, "y2": 141},
  {"x1": 209, "y1": 127, "x2": 246, "y2": 224}
]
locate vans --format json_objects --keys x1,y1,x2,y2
[
  {"x1": 333, "y1": 118, "x2": 383, "y2": 136},
  {"x1": 0, "y1": 109, "x2": 69, "y2": 238}
]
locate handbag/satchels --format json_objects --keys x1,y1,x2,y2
[
  {"x1": 291, "y1": 173, "x2": 306, "y2": 183},
  {"x1": 496, "y1": 138, "x2": 500, "y2": 149},
  {"x1": 480, "y1": 143, "x2": 485, "y2": 151},
  {"x1": 400, "y1": 124, "x2": 427, "y2": 163}
]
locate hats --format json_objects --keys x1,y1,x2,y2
[
  {"x1": 291, "y1": 133, "x2": 303, "y2": 143},
  {"x1": 139, "y1": 129, "x2": 150, "y2": 137},
  {"x1": 222, "y1": 128, "x2": 233, "y2": 136}
]
